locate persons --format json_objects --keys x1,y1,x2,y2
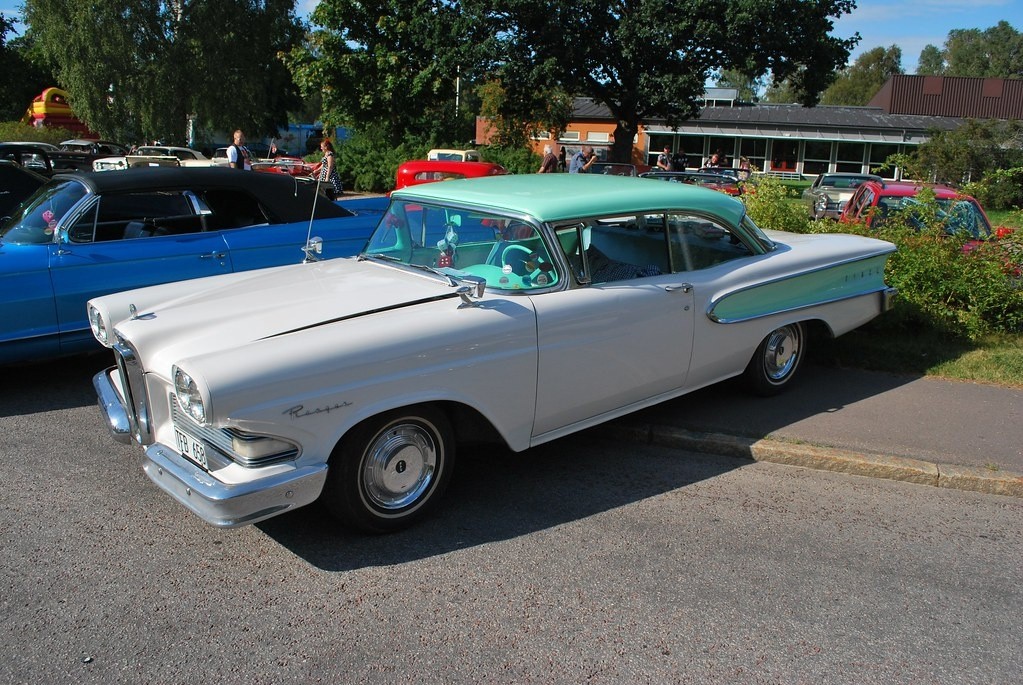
[
  {"x1": 226, "y1": 130, "x2": 254, "y2": 170},
  {"x1": 313, "y1": 139, "x2": 343, "y2": 194},
  {"x1": 569, "y1": 146, "x2": 597, "y2": 173},
  {"x1": 657, "y1": 144, "x2": 689, "y2": 182},
  {"x1": 558, "y1": 147, "x2": 567, "y2": 172},
  {"x1": 703, "y1": 153, "x2": 720, "y2": 183},
  {"x1": 536, "y1": 144, "x2": 557, "y2": 173}
]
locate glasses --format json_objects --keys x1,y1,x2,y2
[{"x1": 237, "y1": 138, "x2": 245, "y2": 141}]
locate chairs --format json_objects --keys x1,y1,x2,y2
[{"x1": 122, "y1": 201, "x2": 154, "y2": 239}]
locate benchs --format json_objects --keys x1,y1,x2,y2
[{"x1": 576, "y1": 226, "x2": 733, "y2": 274}]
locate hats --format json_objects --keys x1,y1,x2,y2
[
  {"x1": 664, "y1": 145, "x2": 671, "y2": 149},
  {"x1": 545, "y1": 147, "x2": 551, "y2": 154}
]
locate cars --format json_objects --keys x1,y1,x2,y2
[
  {"x1": 86, "y1": 172, "x2": 898, "y2": 534},
  {"x1": 0, "y1": 166, "x2": 393, "y2": 368},
  {"x1": 0, "y1": 122, "x2": 356, "y2": 228},
  {"x1": 386, "y1": 148, "x2": 508, "y2": 198},
  {"x1": 586, "y1": 162, "x2": 1023, "y2": 282}
]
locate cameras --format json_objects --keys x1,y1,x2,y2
[{"x1": 590, "y1": 154, "x2": 600, "y2": 160}]
